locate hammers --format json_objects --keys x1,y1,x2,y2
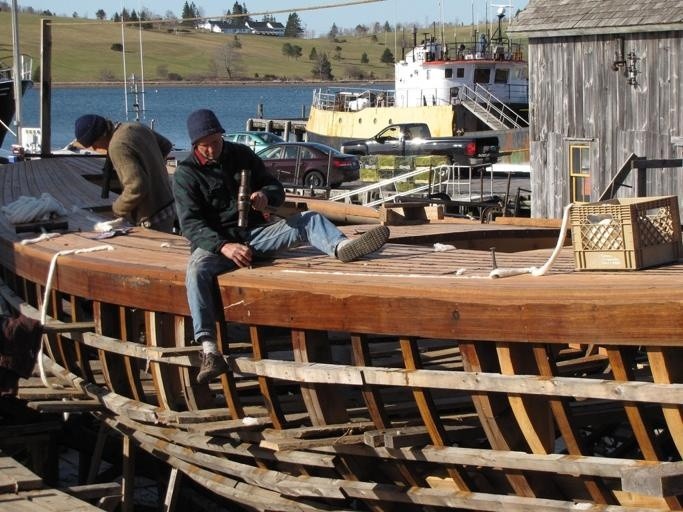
[
  {"x1": 236, "y1": 166, "x2": 298, "y2": 229},
  {"x1": 98, "y1": 156, "x2": 118, "y2": 199}
]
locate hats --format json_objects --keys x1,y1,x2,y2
[
  {"x1": 75, "y1": 114, "x2": 106, "y2": 148},
  {"x1": 187, "y1": 110, "x2": 225, "y2": 144}
]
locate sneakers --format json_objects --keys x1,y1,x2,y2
[
  {"x1": 197, "y1": 351, "x2": 229, "y2": 383},
  {"x1": 339, "y1": 225, "x2": 390, "y2": 262}
]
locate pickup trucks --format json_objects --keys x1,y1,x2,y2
[{"x1": 340, "y1": 123, "x2": 498, "y2": 178}]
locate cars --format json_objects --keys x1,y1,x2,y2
[
  {"x1": 221, "y1": 131, "x2": 284, "y2": 155},
  {"x1": 255, "y1": 142, "x2": 360, "y2": 187}
]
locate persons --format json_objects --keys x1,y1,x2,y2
[
  {"x1": 479, "y1": 34, "x2": 487, "y2": 56},
  {"x1": 174, "y1": 108, "x2": 389, "y2": 382},
  {"x1": 74, "y1": 115, "x2": 175, "y2": 231}
]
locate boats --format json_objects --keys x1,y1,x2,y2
[
  {"x1": 0, "y1": 54, "x2": 33, "y2": 151},
  {"x1": 243, "y1": 8, "x2": 534, "y2": 155}
]
